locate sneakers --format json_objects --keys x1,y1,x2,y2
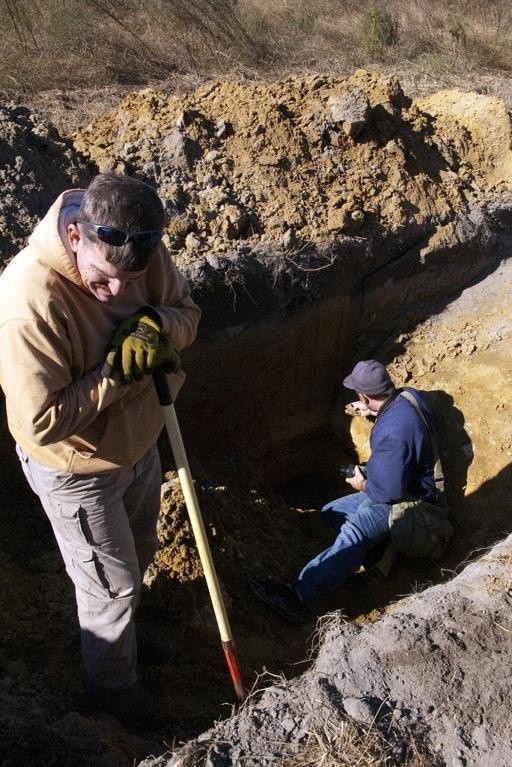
[
  {"x1": 92, "y1": 685, "x2": 160, "y2": 728},
  {"x1": 250, "y1": 576, "x2": 310, "y2": 624}
]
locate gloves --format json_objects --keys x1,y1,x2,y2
[{"x1": 101, "y1": 314, "x2": 181, "y2": 383}]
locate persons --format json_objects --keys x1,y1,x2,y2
[
  {"x1": 0, "y1": 172, "x2": 202, "y2": 727},
  {"x1": 250, "y1": 360, "x2": 439, "y2": 626}
]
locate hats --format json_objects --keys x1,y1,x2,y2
[{"x1": 343, "y1": 361, "x2": 392, "y2": 396}]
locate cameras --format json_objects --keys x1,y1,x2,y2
[{"x1": 336, "y1": 465, "x2": 367, "y2": 479}]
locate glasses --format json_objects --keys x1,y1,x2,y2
[{"x1": 76, "y1": 220, "x2": 163, "y2": 249}]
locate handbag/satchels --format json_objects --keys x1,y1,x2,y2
[{"x1": 389, "y1": 493, "x2": 454, "y2": 563}]
